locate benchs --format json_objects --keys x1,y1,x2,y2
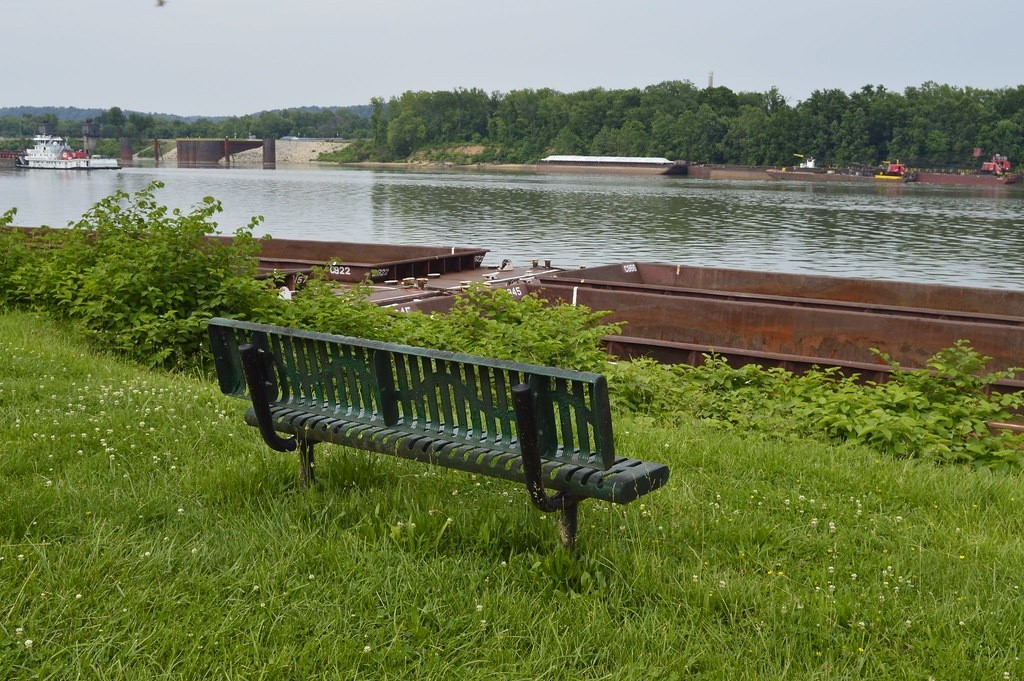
[{"x1": 207, "y1": 319, "x2": 669, "y2": 543}]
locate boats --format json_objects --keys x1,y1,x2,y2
[
  {"x1": 765, "y1": 152, "x2": 918, "y2": 183},
  {"x1": 919, "y1": 146, "x2": 1023, "y2": 186},
  {"x1": 13, "y1": 132, "x2": 121, "y2": 170}
]
